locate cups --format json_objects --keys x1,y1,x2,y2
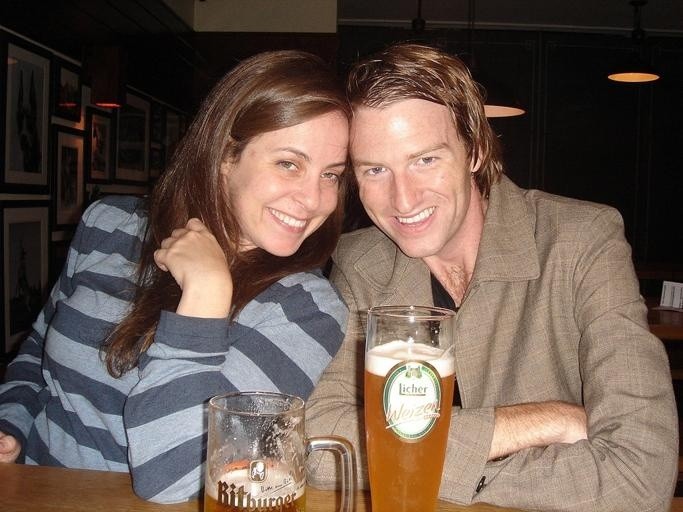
[
  {"x1": 202, "y1": 390, "x2": 359, "y2": 511},
  {"x1": 366, "y1": 304, "x2": 458, "y2": 512}
]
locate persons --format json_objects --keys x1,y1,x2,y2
[
  {"x1": 304, "y1": 44, "x2": 679, "y2": 512},
  {"x1": 0, "y1": 47, "x2": 349, "y2": 503}
]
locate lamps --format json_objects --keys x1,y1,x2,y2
[
  {"x1": 466, "y1": 2, "x2": 526, "y2": 120},
  {"x1": 606, "y1": 2, "x2": 662, "y2": 86}
]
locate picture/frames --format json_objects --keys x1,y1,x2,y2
[{"x1": 0, "y1": 31, "x2": 191, "y2": 363}]
